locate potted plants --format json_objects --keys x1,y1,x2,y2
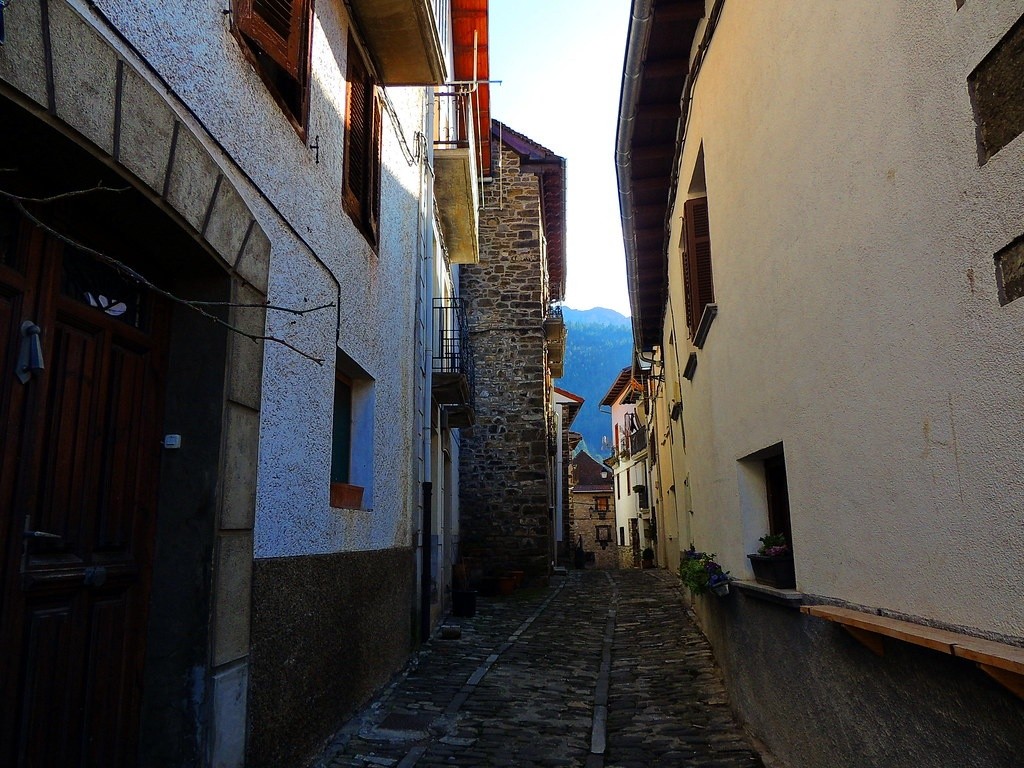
[{"x1": 633, "y1": 547, "x2": 654, "y2": 568}]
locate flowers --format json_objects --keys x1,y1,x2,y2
[
  {"x1": 682, "y1": 541, "x2": 731, "y2": 588},
  {"x1": 759, "y1": 533, "x2": 790, "y2": 556}
]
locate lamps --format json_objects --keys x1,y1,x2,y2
[
  {"x1": 634, "y1": 348, "x2": 664, "y2": 370},
  {"x1": 601, "y1": 467, "x2": 614, "y2": 485}
]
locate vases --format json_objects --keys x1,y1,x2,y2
[
  {"x1": 747, "y1": 553, "x2": 798, "y2": 590},
  {"x1": 711, "y1": 578, "x2": 734, "y2": 598},
  {"x1": 544, "y1": 317, "x2": 564, "y2": 379},
  {"x1": 479, "y1": 562, "x2": 524, "y2": 597},
  {"x1": 330, "y1": 480, "x2": 366, "y2": 512}
]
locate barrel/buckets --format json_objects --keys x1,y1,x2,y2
[
  {"x1": 505, "y1": 571, "x2": 524, "y2": 589},
  {"x1": 499, "y1": 577, "x2": 515, "y2": 595},
  {"x1": 480, "y1": 576, "x2": 496, "y2": 597}
]
